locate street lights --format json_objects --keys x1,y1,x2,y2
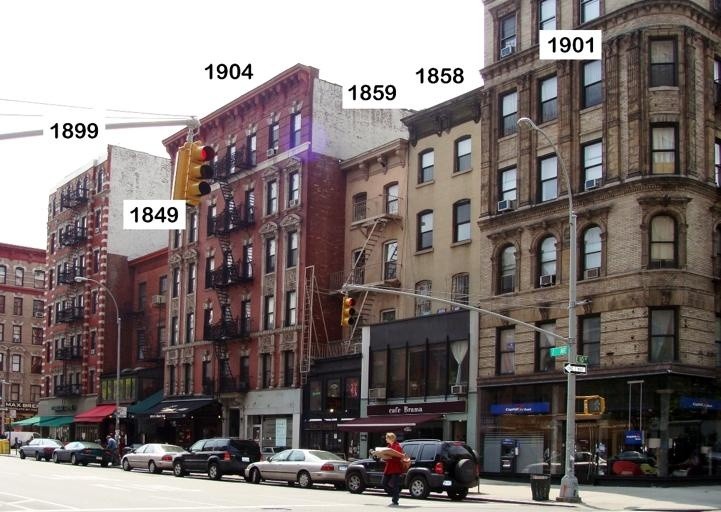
[
  {"x1": 516, "y1": 114, "x2": 581, "y2": 505},
  {"x1": 74, "y1": 275, "x2": 120, "y2": 441}
]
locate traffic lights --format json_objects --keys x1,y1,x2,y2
[
  {"x1": 183, "y1": 143, "x2": 216, "y2": 203},
  {"x1": 342, "y1": 296, "x2": 356, "y2": 327},
  {"x1": 584, "y1": 395, "x2": 606, "y2": 415}
]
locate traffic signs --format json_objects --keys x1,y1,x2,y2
[
  {"x1": 564, "y1": 361, "x2": 589, "y2": 375},
  {"x1": 550, "y1": 346, "x2": 569, "y2": 356},
  {"x1": 576, "y1": 355, "x2": 590, "y2": 365}
]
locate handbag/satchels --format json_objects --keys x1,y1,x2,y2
[{"x1": 400, "y1": 457, "x2": 412, "y2": 470}]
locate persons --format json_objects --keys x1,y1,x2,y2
[
  {"x1": 106, "y1": 434, "x2": 121, "y2": 465},
  {"x1": 375, "y1": 431, "x2": 406, "y2": 505}
]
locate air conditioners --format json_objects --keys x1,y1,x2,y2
[
  {"x1": 540, "y1": 274, "x2": 556, "y2": 286},
  {"x1": 587, "y1": 267, "x2": 599, "y2": 278},
  {"x1": 451, "y1": 385, "x2": 468, "y2": 395},
  {"x1": 369, "y1": 388, "x2": 387, "y2": 399},
  {"x1": 267, "y1": 148, "x2": 277, "y2": 157},
  {"x1": 500, "y1": 45, "x2": 513, "y2": 58},
  {"x1": 585, "y1": 178, "x2": 599, "y2": 189},
  {"x1": 288, "y1": 199, "x2": 302, "y2": 209},
  {"x1": 498, "y1": 199, "x2": 512, "y2": 211}
]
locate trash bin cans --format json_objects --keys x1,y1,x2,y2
[{"x1": 530, "y1": 473, "x2": 551, "y2": 500}]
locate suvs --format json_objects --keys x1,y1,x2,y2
[
  {"x1": 346, "y1": 438, "x2": 482, "y2": 499},
  {"x1": 501, "y1": 447, "x2": 517, "y2": 472},
  {"x1": 174, "y1": 437, "x2": 261, "y2": 480}
]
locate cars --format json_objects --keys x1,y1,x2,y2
[
  {"x1": 242, "y1": 448, "x2": 353, "y2": 488},
  {"x1": 120, "y1": 443, "x2": 191, "y2": 473},
  {"x1": 609, "y1": 450, "x2": 658, "y2": 467},
  {"x1": 526, "y1": 451, "x2": 608, "y2": 475},
  {"x1": 18, "y1": 437, "x2": 65, "y2": 461},
  {"x1": 52, "y1": 441, "x2": 114, "y2": 467}
]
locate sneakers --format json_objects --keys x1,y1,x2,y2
[{"x1": 387, "y1": 502, "x2": 399, "y2": 507}]
